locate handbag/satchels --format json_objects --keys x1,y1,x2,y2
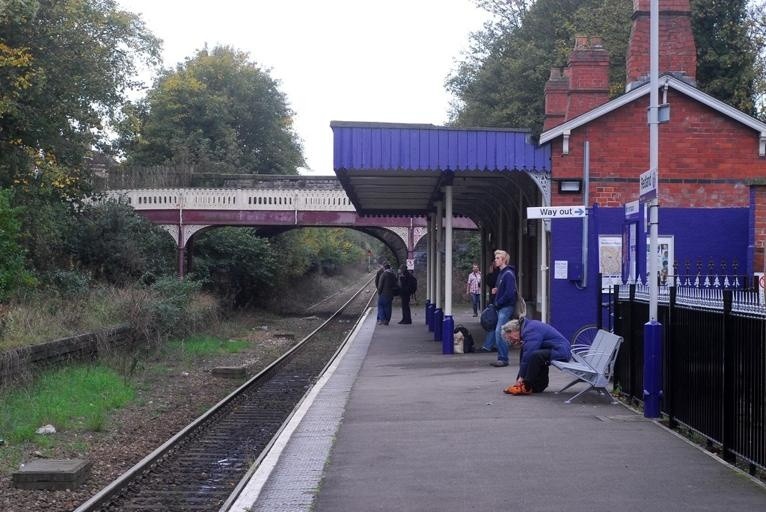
[
  {"x1": 480, "y1": 306, "x2": 497, "y2": 330},
  {"x1": 392, "y1": 286, "x2": 400, "y2": 296},
  {"x1": 513, "y1": 294, "x2": 527, "y2": 318},
  {"x1": 454, "y1": 325, "x2": 474, "y2": 353}
]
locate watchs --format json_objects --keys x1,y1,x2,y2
[{"x1": 375, "y1": 261, "x2": 386, "y2": 322}]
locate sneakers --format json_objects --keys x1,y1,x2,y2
[
  {"x1": 398, "y1": 318, "x2": 411, "y2": 324},
  {"x1": 490, "y1": 360, "x2": 509, "y2": 367},
  {"x1": 479, "y1": 345, "x2": 492, "y2": 353},
  {"x1": 503, "y1": 382, "x2": 533, "y2": 394},
  {"x1": 473, "y1": 312, "x2": 479, "y2": 317}
]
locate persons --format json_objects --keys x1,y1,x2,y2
[
  {"x1": 467, "y1": 262, "x2": 482, "y2": 317},
  {"x1": 397, "y1": 264, "x2": 411, "y2": 324},
  {"x1": 500, "y1": 316, "x2": 573, "y2": 395},
  {"x1": 486, "y1": 258, "x2": 497, "y2": 303},
  {"x1": 475, "y1": 248, "x2": 517, "y2": 368},
  {"x1": 378, "y1": 264, "x2": 396, "y2": 326}
]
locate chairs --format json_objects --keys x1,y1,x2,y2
[{"x1": 549, "y1": 329, "x2": 624, "y2": 406}]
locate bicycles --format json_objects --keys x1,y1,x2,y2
[{"x1": 572, "y1": 300, "x2": 615, "y2": 363}]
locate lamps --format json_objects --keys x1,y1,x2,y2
[{"x1": 557, "y1": 180, "x2": 582, "y2": 195}]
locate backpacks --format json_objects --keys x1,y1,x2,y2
[{"x1": 405, "y1": 274, "x2": 417, "y2": 294}]
